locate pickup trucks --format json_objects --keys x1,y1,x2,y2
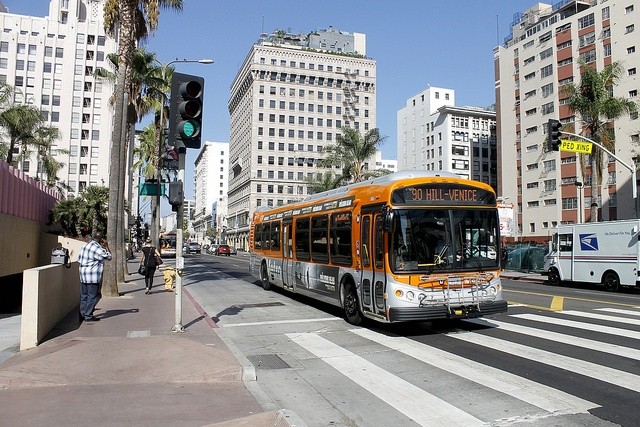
[{"x1": 187, "y1": 242, "x2": 201, "y2": 254}]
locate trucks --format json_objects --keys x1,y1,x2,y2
[{"x1": 540, "y1": 221, "x2": 639, "y2": 293}]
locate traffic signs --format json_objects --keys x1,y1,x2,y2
[{"x1": 560, "y1": 139, "x2": 592, "y2": 154}]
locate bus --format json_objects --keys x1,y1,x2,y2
[
  {"x1": 247, "y1": 169, "x2": 515, "y2": 331},
  {"x1": 158, "y1": 232, "x2": 176, "y2": 257},
  {"x1": 150, "y1": 58, "x2": 216, "y2": 268}
]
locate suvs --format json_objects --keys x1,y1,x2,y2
[
  {"x1": 215, "y1": 243, "x2": 230, "y2": 255},
  {"x1": 207, "y1": 243, "x2": 218, "y2": 254}
]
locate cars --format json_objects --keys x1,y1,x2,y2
[{"x1": 228, "y1": 246, "x2": 237, "y2": 254}]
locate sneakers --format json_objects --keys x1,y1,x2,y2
[{"x1": 85, "y1": 317, "x2": 100, "y2": 322}]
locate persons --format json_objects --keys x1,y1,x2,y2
[
  {"x1": 140, "y1": 237, "x2": 160, "y2": 294},
  {"x1": 435, "y1": 232, "x2": 458, "y2": 260},
  {"x1": 501, "y1": 246, "x2": 508, "y2": 271},
  {"x1": 77, "y1": 231, "x2": 112, "y2": 321}
]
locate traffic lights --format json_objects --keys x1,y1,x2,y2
[
  {"x1": 167, "y1": 69, "x2": 202, "y2": 149},
  {"x1": 547, "y1": 118, "x2": 563, "y2": 153}
]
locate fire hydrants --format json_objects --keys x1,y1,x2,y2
[{"x1": 160, "y1": 265, "x2": 176, "y2": 292}]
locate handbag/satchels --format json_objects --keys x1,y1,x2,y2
[
  {"x1": 50, "y1": 247, "x2": 68, "y2": 264},
  {"x1": 154, "y1": 256, "x2": 163, "y2": 266},
  {"x1": 138, "y1": 265, "x2": 149, "y2": 276}
]
